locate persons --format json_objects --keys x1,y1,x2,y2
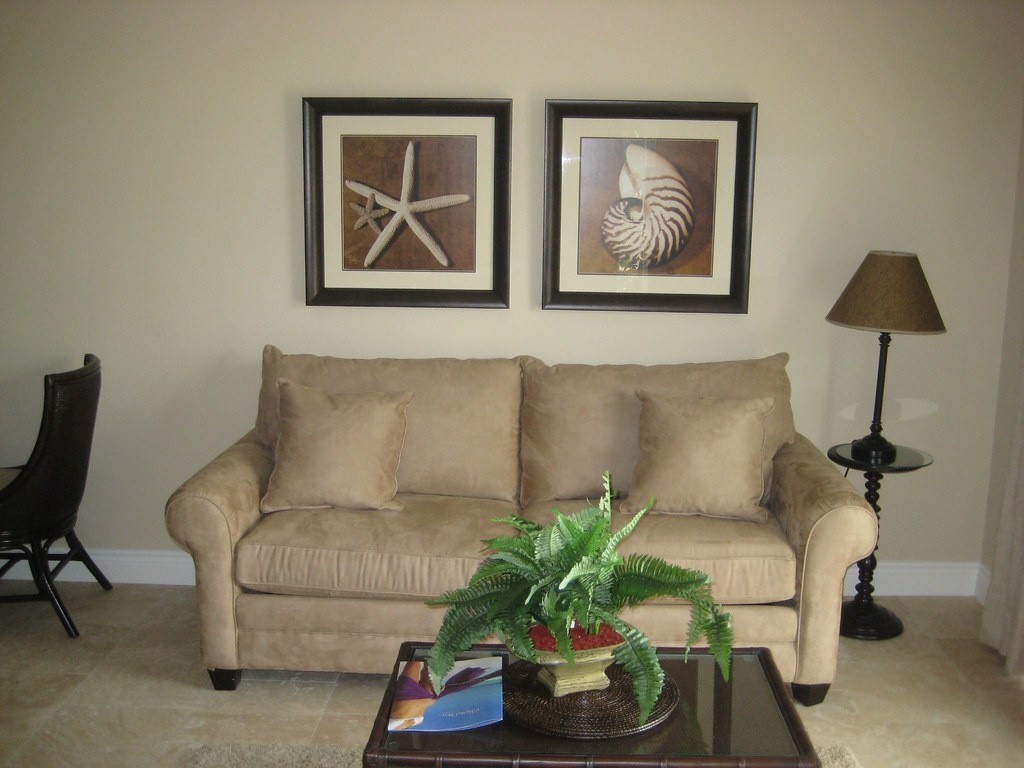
[{"x1": 388, "y1": 661, "x2": 440, "y2": 731}]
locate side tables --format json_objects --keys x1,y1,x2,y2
[{"x1": 827, "y1": 444, "x2": 933, "y2": 641}]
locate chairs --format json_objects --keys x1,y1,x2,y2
[{"x1": 0, "y1": 354, "x2": 114, "y2": 638}]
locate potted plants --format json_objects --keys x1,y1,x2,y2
[{"x1": 424, "y1": 467, "x2": 734, "y2": 725}]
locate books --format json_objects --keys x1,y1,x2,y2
[{"x1": 388, "y1": 657, "x2": 504, "y2": 732}]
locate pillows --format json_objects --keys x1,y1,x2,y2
[
  {"x1": 619, "y1": 391, "x2": 776, "y2": 524},
  {"x1": 260, "y1": 378, "x2": 414, "y2": 514}
]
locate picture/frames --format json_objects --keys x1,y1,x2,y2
[
  {"x1": 302, "y1": 96, "x2": 513, "y2": 308},
  {"x1": 541, "y1": 100, "x2": 758, "y2": 314}
]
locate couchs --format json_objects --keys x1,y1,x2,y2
[{"x1": 165, "y1": 343, "x2": 878, "y2": 707}]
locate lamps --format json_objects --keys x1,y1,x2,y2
[{"x1": 825, "y1": 250, "x2": 947, "y2": 462}]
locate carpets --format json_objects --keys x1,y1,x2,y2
[{"x1": 183, "y1": 742, "x2": 862, "y2": 768}]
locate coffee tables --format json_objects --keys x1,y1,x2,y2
[{"x1": 362, "y1": 641, "x2": 823, "y2": 768}]
